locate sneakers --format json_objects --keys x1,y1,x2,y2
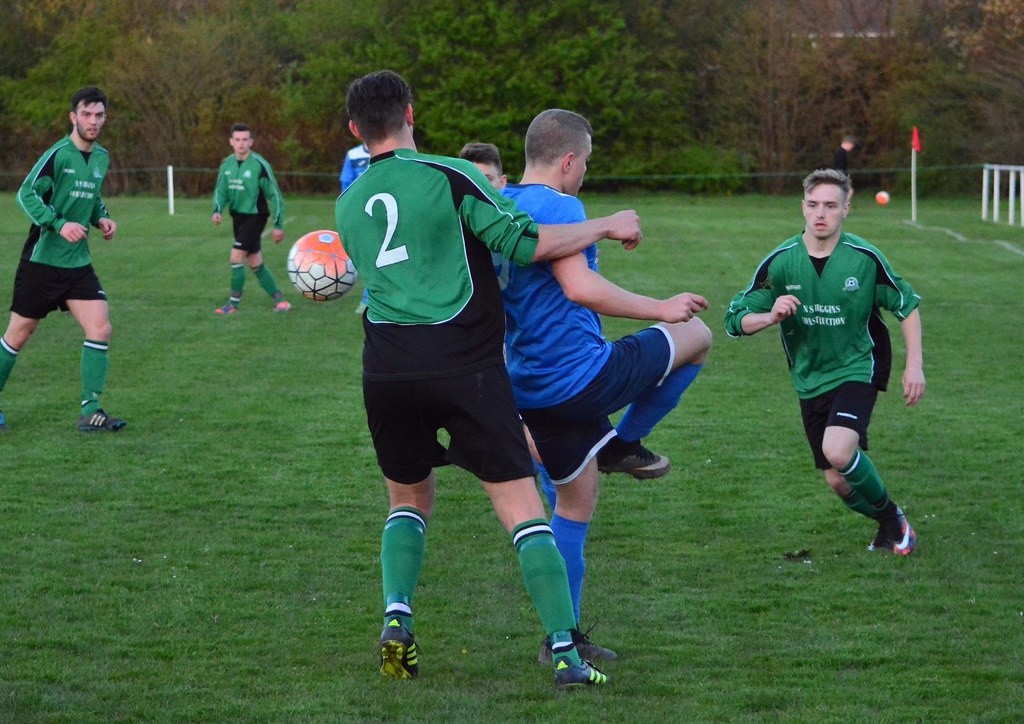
[
  {"x1": 214, "y1": 303, "x2": 237, "y2": 314},
  {"x1": 271, "y1": 301, "x2": 294, "y2": 311},
  {"x1": 867, "y1": 502, "x2": 917, "y2": 555},
  {"x1": 554, "y1": 658, "x2": 615, "y2": 688},
  {"x1": 78, "y1": 407, "x2": 126, "y2": 432},
  {"x1": 595, "y1": 434, "x2": 671, "y2": 480},
  {"x1": 355, "y1": 301, "x2": 368, "y2": 314},
  {"x1": 377, "y1": 614, "x2": 422, "y2": 679},
  {"x1": 538, "y1": 622, "x2": 619, "y2": 662}
]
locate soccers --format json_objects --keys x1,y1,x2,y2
[
  {"x1": 875, "y1": 190, "x2": 890, "y2": 206},
  {"x1": 286, "y1": 229, "x2": 360, "y2": 303}
]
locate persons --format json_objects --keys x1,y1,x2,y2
[
  {"x1": 723, "y1": 168, "x2": 926, "y2": 555},
  {"x1": 333, "y1": 69, "x2": 643, "y2": 687},
  {"x1": 212, "y1": 124, "x2": 290, "y2": 314},
  {"x1": 459, "y1": 109, "x2": 713, "y2": 663},
  {"x1": 339, "y1": 143, "x2": 371, "y2": 313},
  {"x1": 0, "y1": 87, "x2": 126, "y2": 432}
]
